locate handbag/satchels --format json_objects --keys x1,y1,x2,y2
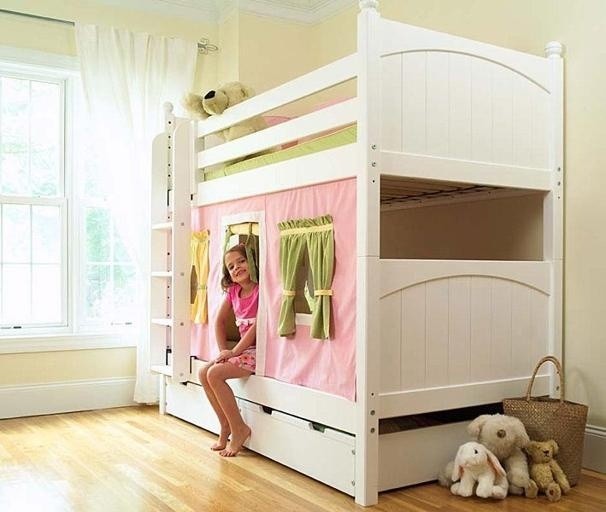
[{"x1": 503, "y1": 357, "x2": 589, "y2": 487}]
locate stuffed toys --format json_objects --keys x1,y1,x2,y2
[
  {"x1": 450, "y1": 441, "x2": 509, "y2": 500},
  {"x1": 438, "y1": 413, "x2": 531, "y2": 496},
  {"x1": 181, "y1": 80, "x2": 279, "y2": 167},
  {"x1": 524, "y1": 439, "x2": 571, "y2": 503}
]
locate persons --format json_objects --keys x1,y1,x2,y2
[{"x1": 199, "y1": 245, "x2": 259, "y2": 457}]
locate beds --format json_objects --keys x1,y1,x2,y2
[{"x1": 147, "y1": 1, "x2": 564, "y2": 506}]
